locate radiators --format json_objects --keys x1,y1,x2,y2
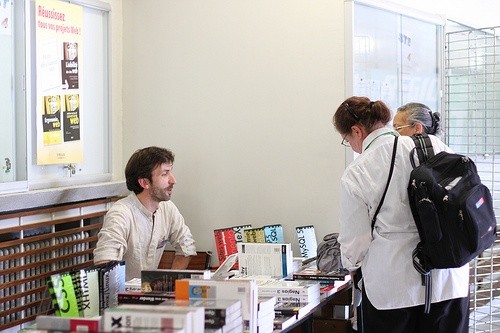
[{"x1": 0, "y1": 232, "x2": 89, "y2": 328}]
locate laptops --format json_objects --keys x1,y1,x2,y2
[{"x1": 211, "y1": 253, "x2": 239, "y2": 278}]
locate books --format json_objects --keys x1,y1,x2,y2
[{"x1": 14, "y1": 224, "x2": 352, "y2": 333}]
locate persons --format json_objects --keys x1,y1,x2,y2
[
  {"x1": 333, "y1": 96, "x2": 471, "y2": 333},
  {"x1": 93, "y1": 146, "x2": 197, "y2": 282},
  {"x1": 392, "y1": 102, "x2": 440, "y2": 137}
]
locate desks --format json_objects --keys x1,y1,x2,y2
[{"x1": 17, "y1": 257, "x2": 360, "y2": 333}]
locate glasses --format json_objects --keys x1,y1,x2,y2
[{"x1": 341, "y1": 123, "x2": 359, "y2": 147}]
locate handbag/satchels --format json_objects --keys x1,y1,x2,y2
[{"x1": 317, "y1": 234, "x2": 350, "y2": 276}]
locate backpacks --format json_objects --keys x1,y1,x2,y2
[{"x1": 407, "y1": 131, "x2": 498, "y2": 276}]
